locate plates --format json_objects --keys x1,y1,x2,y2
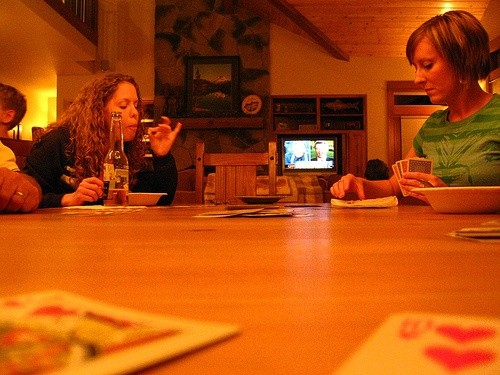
[{"x1": 235, "y1": 195, "x2": 286, "y2": 204}]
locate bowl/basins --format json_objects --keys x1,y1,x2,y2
[
  {"x1": 411, "y1": 186, "x2": 500, "y2": 213},
  {"x1": 129, "y1": 193, "x2": 167, "y2": 205}
]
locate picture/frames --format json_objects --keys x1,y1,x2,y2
[{"x1": 185, "y1": 55, "x2": 239, "y2": 117}]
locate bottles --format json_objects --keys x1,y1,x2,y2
[{"x1": 103, "y1": 112, "x2": 128, "y2": 206}]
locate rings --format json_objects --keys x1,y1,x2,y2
[
  {"x1": 338, "y1": 180, "x2": 342, "y2": 183},
  {"x1": 14, "y1": 191, "x2": 25, "y2": 198}
]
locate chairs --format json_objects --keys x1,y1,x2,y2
[{"x1": 194, "y1": 142, "x2": 278, "y2": 205}]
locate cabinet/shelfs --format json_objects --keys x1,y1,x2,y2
[{"x1": 272, "y1": 94, "x2": 368, "y2": 201}]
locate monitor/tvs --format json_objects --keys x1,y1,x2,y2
[{"x1": 281, "y1": 137, "x2": 338, "y2": 175}]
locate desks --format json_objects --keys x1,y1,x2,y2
[{"x1": 0, "y1": 205, "x2": 500, "y2": 375}]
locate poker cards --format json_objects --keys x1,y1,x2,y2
[
  {"x1": 391, "y1": 157, "x2": 433, "y2": 196},
  {"x1": 442, "y1": 217, "x2": 500, "y2": 245},
  {"x1": 193, "y1": 206, "x2": 316, "y2": 218}
]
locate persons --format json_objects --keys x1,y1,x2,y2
[
  {"x1": 284, "y1": 140, "x2": 334, "y2": 167},
  {"x1": 0, "y1": 83, "x2": 42, "y2": 214},
  {"x1": 329, "y1": 10, "x2": 500, "y2": 206},
  {"x1": 23, "y1": 70, "x2": 182, "y2": 208}
]
